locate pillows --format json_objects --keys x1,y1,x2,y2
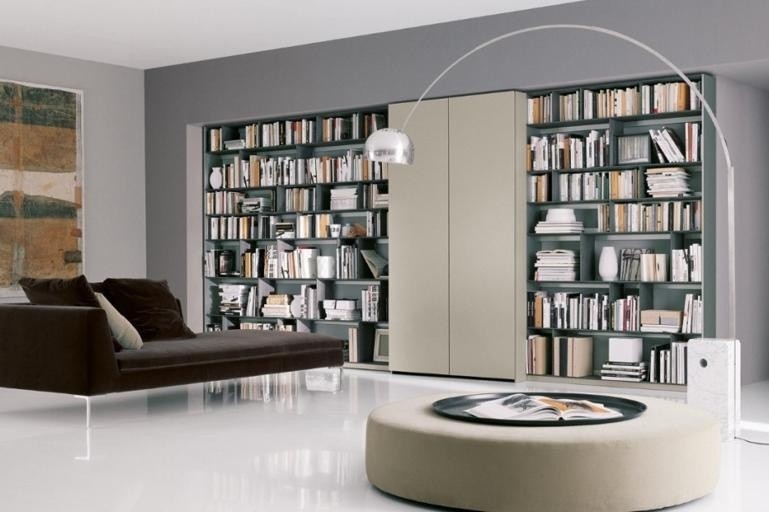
[
  {"x1": 94, "y1": 292, "x2": 144, "y2": 350},
  {"x1": 18, "y1": 275, "x2": 123, "y2": 353},
  {"x1": 102, "y1": 278, "x2": 197, "y2": 341}
]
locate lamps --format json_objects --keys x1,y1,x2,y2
[{"x1": 362, "y1": 23, "x2": 742, "y2": 476}]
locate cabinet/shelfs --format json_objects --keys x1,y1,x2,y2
[
  {"x1": 389, "y1": 89, "x2": 527, "y2": 383},
  {"x1": 527, "y1": 71, "x2": 716, "y2": 392},
  {"x1": 203, "y1": 103, "x2": 389, "y2": 370}
]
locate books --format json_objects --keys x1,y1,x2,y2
[
  {"x1": 618, "y1": 123, "x2": 701, "y2": 166},
  {"x1": 671, "y1": 340, "x2": 688, "y2": 385},
  {"x1": 526, "y1": 335, "x2": 551, "y2": 374},
  {"x1": 463, "y1": 393, "x2": 624, "y2": 420},
  {"x1": 219, "y1": 157, "x2": 240, "y2": 188},
  {"x1": 527, "y1": 290, "x2": 608, "y2": 331},
  {"x1": 343, "y1": 326, "x2": 358, "y2": 362},
  {"x1": 241, "y1": 155, "x2": 295, "y2": 186},
  {"x1": 205, "y1": 248, "x2": 236, "y2": 278},
  {"x1": 598, "y1": 203, "x2": 611, "y2": 232},
  {"x1": 528, "y1": 92, "x2": 550, "y2": 125},
  {"x1": 321, "y1": 298, "x2": 361, "y2": 322},
  {"x1": 241, "y1": 245, "x2": 278, "y2": 279},
  {"x1": 528, "y1": 129, "x2": 610, "y2": 172},
  {"x1": 335, "y1": 245, "x2": 357, "y2": 280},
  {"x1": 553, "y1": 337, "x2": 594, "y2": 377},
  {"x1": 207, "y1": 322, "x2": 221, "y2": 332},
  {"x1": 558, "y1": 91, "x2": 580, "y2": 121},
  {"x1": 207, "y1": 216, "x2": 282, "y2": 240},
  {"x1": 297, "y1": 215, "x2": 334, "y2": 238},
  {"x1": 614, "y1": 202, "x2": 701, "y2": 232},
  {"x1": 584, "y1": 85, "x2": 651, "y2": 119},
  {"x1": 284, "y1": 187, "x2": 316, "y2": 211},
  {"x1": 645, "y1": 168, "x2": 694, "y2": 197},
  {"x1": 612, "y1": 294, "x2": 639, "y2": 331},
  {"x1": 557, "y1": 169, "x2": 639, "y2": 200},
  {"x1": 244, "y1": 125, "x2": 259, "y2": 148},
  {"x1": 363, "y1": 184, "x2": 389, "y2": 208},
  {"x1": 534, "y1": 248, "x2": 580, "y2": 282},
  {"x1": 649, "y1": 345, "x2": 671, "y2": 383},
  {"x1": 322, "y1": 114, "x2": 387, "y2": 141},
  {"x1": 210, "y1": 128, "x2": 222, "y2": 151},
  {"x1": 600, "y1": 361, "x2": 647, "y2": 382},
  {"x1": 671, "y1": 243, "x2": 702, "y2": 283},
  {"x1": 240, "y1": 322, "x2": 295, "y2": 332},
  {"x1": 619, "y1": 249, "x2": 656, "y2": 283},
  {"x1": 216, "y1": 283, "x2": 256, "y2": 316},
  {"x1": 225, "y1": 139, "x2": 245, "y2": 149},
  {"x1": 361, "y1": 285, "x2": 379, "y2": 322},
  {"x1": 528, "y1": 173, "x2": 548, "y2": 202},
  {"x1": 298, "y1": 151, "x2": 352, "y2": 183},
  {"x1": 533, "y1": 221, "x2": 584, "y2": 233},
  {"x1": 299, "y1": 283, "x2": 317, "y2": 319},
  {"x1": 354, "y1": 153, "x2": 388, "y2": 181},
  {"x1": 279, "y1": 245, "x2": 320, "y2": 279},
  {"x1": 262, "y1": 119, "x2": 316, "y2": 147},
  {"x1": 204, "y1": 191, "x2": 244, "y2": 216},
  {"x1": 260, "y1": 294, "x2": 293, "y2": 319},
  {"x1": 641, "y1": 294, "x2": 701, "y2": 335},
  {"x1": 655, "y1": 82, "x2": 700, "y2": 112},
  {"x1": 331, "y1": 185, "x2": 357, "y2": 209},
  {"x1": 366, "y1": 211, "x2": 387, "y2": 237}
]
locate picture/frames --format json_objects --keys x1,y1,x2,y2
[
  {"x1": 373, "y1": 329, "x2": 389, "y2": 362},
  {"x1": 0, "y1": 80, "x2": 85, "y2": 303}
]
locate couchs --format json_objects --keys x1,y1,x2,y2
[
  {"x1": 0, "y1": 275, "x2": 344, "y2": 426},
  {"x1": 369, "y1": 387, "x2": 718, "y2": 512}
]
